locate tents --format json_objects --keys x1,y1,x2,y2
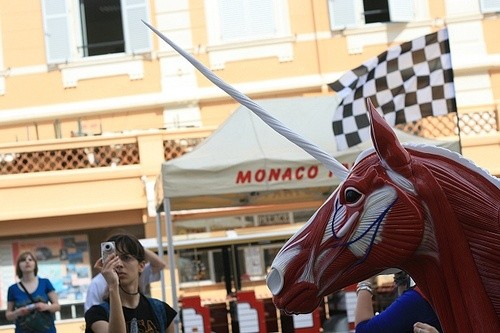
[{"x1": 155, "y1": 95, "x2": 462, "y2": 332}]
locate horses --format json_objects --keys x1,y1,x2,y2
[{"x1": 266, "y1": 96, "x2": 500, "y2": 333}]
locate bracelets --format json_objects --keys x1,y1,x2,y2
[{"x1": 355, "y1": 279, "x2": 374, "y2": 295}]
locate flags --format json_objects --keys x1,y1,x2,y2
[{"x1": 324, "y1": 25, "x2": 457, "y2": 150}]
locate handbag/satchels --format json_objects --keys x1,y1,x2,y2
[{"x1": 15, "y1": 299, "x2": 56, "y2": 333}]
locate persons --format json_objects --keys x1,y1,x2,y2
[
  {"x1": 353, "y1": 271, "x2": 443, "y2": 333},
  {"x1": 84, "y1": 233, "x2": 177, "y2": 333},
  {"x1": 6, "y1": 251, "x2": 60, "y2": 333}
]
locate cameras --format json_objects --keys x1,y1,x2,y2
[{"x1": 101, "y1": 242, "x2": 116, "y2": 263}]
